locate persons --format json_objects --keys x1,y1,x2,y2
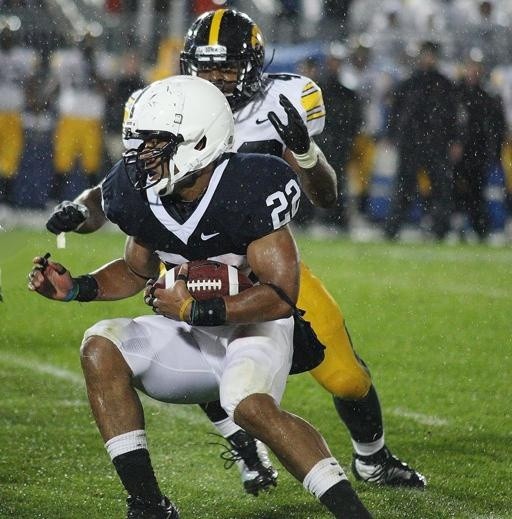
[
  {"x1": 25, "y1": 73, "x2": 381, "y2": 519},
  {"x1": 44, "y1": 7, "x2": 432, "y2": 489},
  {"x1": 0, "y1": 0, "x2": 512, "y2": 246}
]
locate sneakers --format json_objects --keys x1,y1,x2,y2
[
  {"x1": 124, "y1": 494, "x2": 180, "y2": 518},
  {"x1": 353, "y1": 444, "x2": 426, "y2": 489},
  {"x1": 222, "y1": 428, "x2": 278, "y2": 494}
]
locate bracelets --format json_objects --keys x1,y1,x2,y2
[
  {"x1": 71, "y1": 272, "x2": 100, "y2": 306},
  {"x1": 186, "y1": 291, "x2": 227, "y2": 329},
  {"x1": 174, "y1": 293, "x2": 195, "y2": 324}
]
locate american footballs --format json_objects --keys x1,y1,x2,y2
[{"x1": 151, "y1": 260, "x2": 254, "y2": 302}]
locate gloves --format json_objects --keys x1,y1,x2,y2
[
  {"x1": 46, "y1": 200, "x2": 91, "y2": 236},
  {"x1": 268, "y1": 94, "x2": 310, "y2": 155}
]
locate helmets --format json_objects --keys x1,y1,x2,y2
[
  {"x1": 123, "y1": 74, "x2": 233, "y2": 207},
  {"x1": 179, "y1": 9, "x2": 265, "y2": 112}
]
